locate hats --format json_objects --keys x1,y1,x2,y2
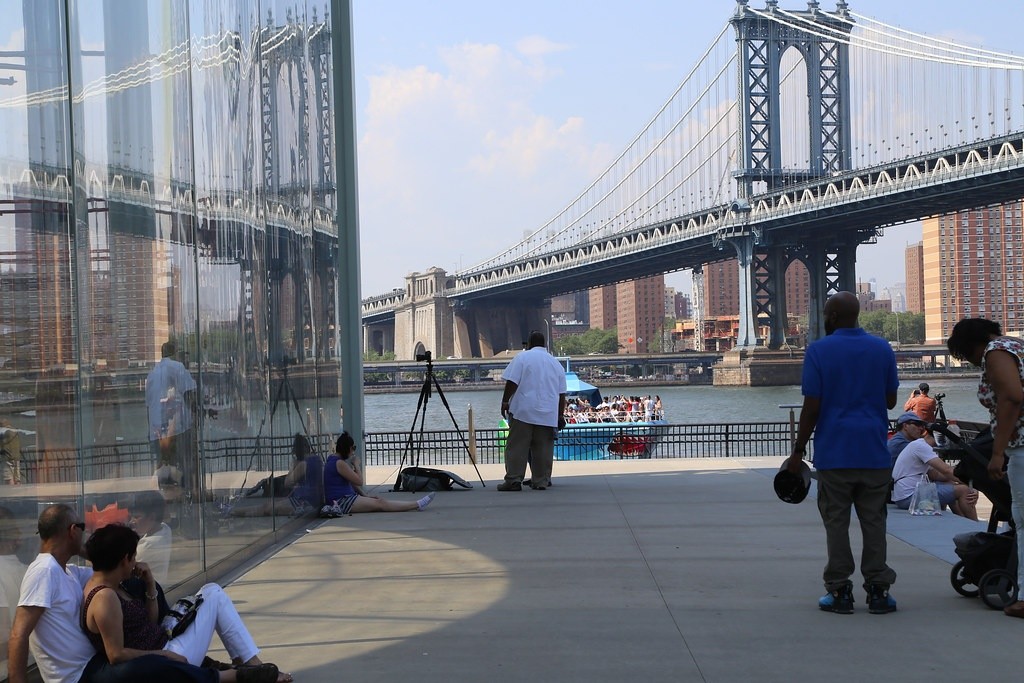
[
  {"x1": 919, "y1": 383, "x2": 929, "y2": 390},
  {"x1": 897, "y1": 412, "x2": 928, "y2": 426},
  {"x1": 774, "y1": 458, "x2": 811, "y2": 504}
]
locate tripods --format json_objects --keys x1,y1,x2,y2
[
  {"x1": 935, "y1": 400, "x2": 946, "y2": 419},
  {"x1": 238, "y1": 367, "x2": 317, "y2": 492},
  {"x1": 395, "y1": 360, "x2": 486, "y2": 487}
]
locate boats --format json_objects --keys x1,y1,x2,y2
[{"x1": 505, "y1": 357, "x2": 673, "y2": 461}]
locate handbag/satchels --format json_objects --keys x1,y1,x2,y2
[
  {"x1": 908, "y1": 474, "x2": 943, "y2": 516},
  {"x1": 389, "y1": 467, "x2": 473, "y2": 493}
]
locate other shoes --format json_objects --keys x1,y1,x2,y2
[
  {"x1": 200, "y1": 656, "x2": 232, "y2": 671},
  {"x1": 417, "y1": 492, "x2": 435, "y2": 512},
  {"x1": 523, "y1": 479, "x2": 552, "y2": 490},
  {"x1": 233, "y1": 662, "x2": 279, "y2": 683},
  {"x1": 497, "y1": 482, "x2": 522, "y2": 491},
  {"x1": 1004, "y1": 600, "x2": 1024, "y2": 618}
]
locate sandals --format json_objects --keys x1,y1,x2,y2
[{"x1": 277, "y1": 671, "x2": 294, "y2": 683}]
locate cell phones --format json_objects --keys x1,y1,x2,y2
[{"x1": 914, "y1": 390, "x2": 920, "y2": 394}]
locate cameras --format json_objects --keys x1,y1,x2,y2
[
  {"x1": 416, "y1": 351, "x2": 431, "y2": 361},
  {"x1": 935, "y1": 393, "x2": 945, "y2": 400},
  {"x1": 283, "y1": 355, "x2": 298, "y2": 367}
]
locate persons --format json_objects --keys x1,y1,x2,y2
[
  {"x1": 0, "y1": 507, "x2": 30, "y2": 662},
  {"x1": 145, "y1": 341, "x2": 220, "y2": 504},
  {"x1": 787, "y1": 291, "x2": 900, "y2": 614},
  {"x1": 496, "y1": 329, "x2": 567, "y2": 491},
  {"x1": 79, "y1": 521, "x2": 294, "y2": 683},
  {"x1": 8, "y1": 504, "x2": 279, "y2": 683},
  {"x1": 214, "y1": 433, "x2": 324, "y2": 517},
  {"x1": 563, "y1": 395, "x2": 662, "y2": 424},
  {"x1": 323, "y1": 430, "x2": 436, "y2": 513},
  {"x1": 904, "y1": 383, "x2": 937, "y2": 423},
  {"x1": 946, "y1": 318, "x2": 1024, "y2": 619},
  {"x1": 890, "y1": 423, "x2": 979, "y2": 521},
  {"x1": 0, "y1": 418, "x2": 21, "y2": 485},
  {"x1": 127, "y1": 490, "x2": 172, "y2": 591},
  {"x1": 886, "y1": 411, "x2": 929, "y2": 504}
]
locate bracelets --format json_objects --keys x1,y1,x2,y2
[
  {"x1": 793, "y1": 442, "x2": 807, "y2": 456},
  {"x1": 145, "y1": 589, "x2": 158, "y2": 600},
  {"x1": 502, "y1": 402, "x2": 508, "y2": 404}
]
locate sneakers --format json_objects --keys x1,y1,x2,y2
[
  {"x1": 818, "y1": 583, "x2": 855, "y2": 614},
  {"x1": 868, "y1": 584, "x2": 897, "y2": 613}
]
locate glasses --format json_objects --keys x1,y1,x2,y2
[
  {"x1": 352, "y1": 446, "x2": 356, "y2": 451},
  {"x1": 36, "y1": 522, "x2": 85, "y2": 534}
]
